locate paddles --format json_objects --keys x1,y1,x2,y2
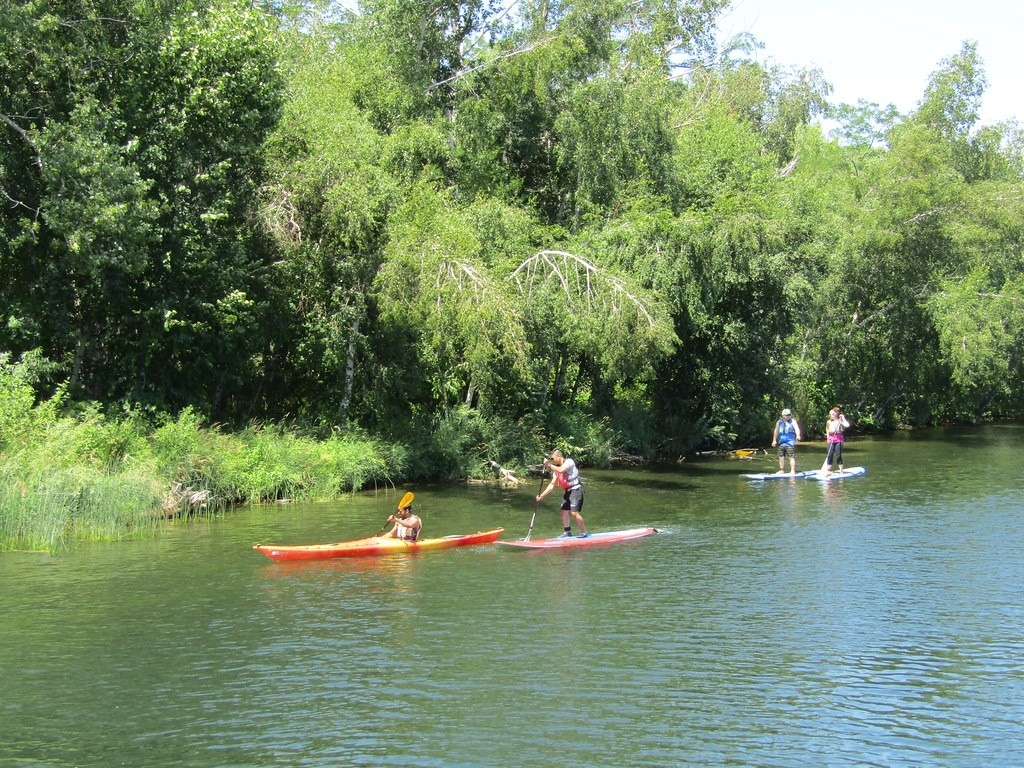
[
  {"x1": 523, "y1": 453, "x2": 550, "y2": 541},
  {"x1": 736, "y1": 438, "x2": 796, "y2": 458},
  {"x1": 374, "y1": 492, "x2": 414, "y2": 539},
  {"x1": 817, "y1": 415, "x2": 842, "y2": 478}
]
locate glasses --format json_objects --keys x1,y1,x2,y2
[
  {"x1": 786, "y1": 414, "x2": 791, "y2": 416},
  {"x1": 548, "y1": 458, "x2": 554, "y2": 460}
]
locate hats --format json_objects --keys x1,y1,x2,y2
[{"x1": 782, "y1": 409, "x2": 791, "y2": 416}]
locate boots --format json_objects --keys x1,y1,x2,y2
[
  {"x1": 826, "y1": 465, "x2": 833, "y2": 476},
  {"x1": 838, "y1": 465, "x2": 844, "y2": 475}
]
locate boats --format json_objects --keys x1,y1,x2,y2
[
  {"x1": 252, "y1": 527, "x2": 505, "y2": 563},
  {"x1": 739, "y1": 467, "x2": 820, "y2": 480},
  {"x1": 805, "y1": 467, "x2": 865, "y2": 481},
  {"x1": 492, "y1": 528, "x2": 658, "y2": 549}
]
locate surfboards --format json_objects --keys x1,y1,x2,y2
[
  {"x1": 492, "y1": 527, "x2": 655, "y2": 549},
  {"x1": 738, "y1": 469, "x2": 820, "y2": 480},
  {"x1": 805, "y1": 466, "x2": 867, "y2": 481}
]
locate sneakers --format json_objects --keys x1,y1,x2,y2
[
  {"x1": 558, "y1": 532, "x2": 572, "y2": 538},
  {"x1": 576, "y1": 532, "x2": 587, "y2": 538}
]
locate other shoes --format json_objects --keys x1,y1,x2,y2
[
  {"x1": 789, "y1": 471, "x2": 796, "y2": 475},
  {"x1": 776, "y1": 471, "x2": 785, "y2": 474}
]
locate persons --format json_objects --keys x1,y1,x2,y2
[
  {"x1": 388, "y1": 502, "x2": 422, "y2": 542},
  {"x1": 536, "y1": 447, "x2": 587, "y2": 540},
  {"x1": 772, "y1": 409, "x2": 801, "y2": 475},
  {"x1": 825, "y1": 407, "x2": 850, "y2": 474}
]
